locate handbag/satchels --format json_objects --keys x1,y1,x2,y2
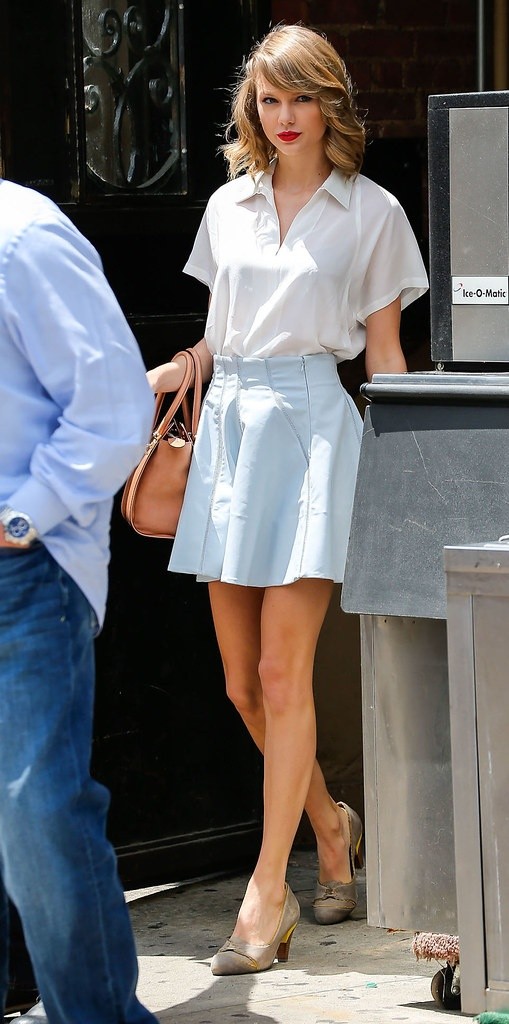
[{"x1": 121, "y1": 347, "x2": 201, "y2": 540}]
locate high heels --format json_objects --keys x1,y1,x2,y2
[
  {"x1": 313, "y1": 801, "x2": 363, "y2": 925},
  {"x1": 211, "y1": 882, "x2": 300, "y2": 974}
]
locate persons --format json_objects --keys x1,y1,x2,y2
[
  {"x1": 0, "y1": 132, "x2": 161, "y2": 1024},
  {"x1": 146, "y1": 19, "x2": 429, "y2": 976}
]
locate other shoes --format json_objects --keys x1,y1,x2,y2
[{"x1": 0, "y1": 985, "x2": 40, "y2": 1015}]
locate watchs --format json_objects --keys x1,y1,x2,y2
[{"x1": 0, "y1": 505, "x2": 39, "y2": 545}]
